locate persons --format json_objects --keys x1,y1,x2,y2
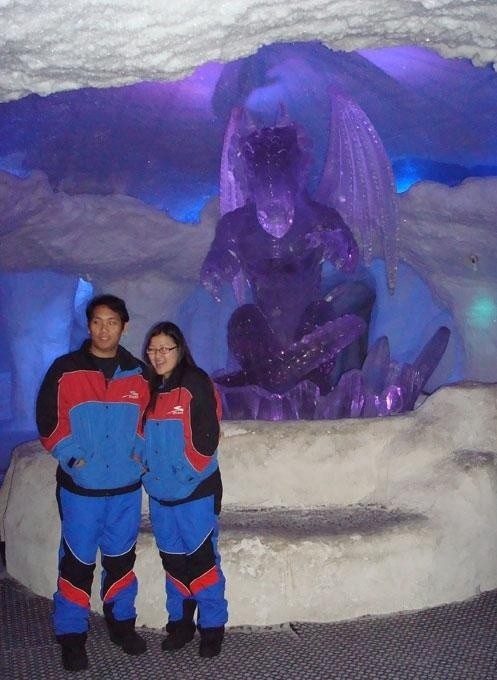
[
  {"x1": 134, "y1": 319, "x2": 229, "y2": 659},
  {"x1": 34, "y1": 293, "x2": 155, "y2": 673}
]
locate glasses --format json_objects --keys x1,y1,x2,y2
[{"x1": 146, "y1": 345, "x2": 178, "y2": 354}]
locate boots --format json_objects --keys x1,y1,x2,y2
[
  {"x1": 162, "y1": 620, "x2": 196, "y2": 651},
  {"x1": 109, "y1": 618, "x2": 146, "y2": 655},
  {"x1": 57, "y1": 633, "x2": 88, "y2": 670},
  {"x1": 199, "y1": 625, "x2": 224, "y2": 658}
]
